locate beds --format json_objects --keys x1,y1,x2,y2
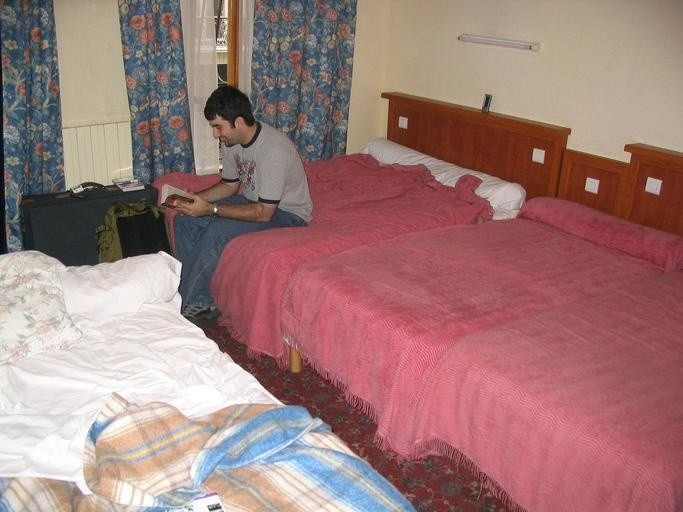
[
  {"x1": 0, "y1": 290, "x2": 419, "y2": 512},
  {"x1": 412, "y1": 268, "x2": 683, "y2": 511},
  {"x1": 277, "y1": 142, "x2": 682, "y2": 468},
  {"x1": 150, "y1": 92, "x2": 570, "y2": 370}
]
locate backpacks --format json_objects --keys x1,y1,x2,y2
[{"x1": 94, "y1": 198, "x2": 174, "y2": 264}]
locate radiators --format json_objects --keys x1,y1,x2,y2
[{"x1": 62, "y1": 117, "x2": 133, "y2": 191}]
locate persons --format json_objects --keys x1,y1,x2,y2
[{"x1": 173, "y1": 86, "x2": 313, "y2": 332}]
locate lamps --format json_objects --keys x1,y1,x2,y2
[{"x1": 457, "y1": 33, "x2": 540, "y2": 51}]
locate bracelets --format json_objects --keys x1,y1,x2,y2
[{"x1": 211, "y1": 203, "x2": 218, "y2": 219}]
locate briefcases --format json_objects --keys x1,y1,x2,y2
[{"x1": 22, "y1": 181, "x2": 158, "y2": 266}]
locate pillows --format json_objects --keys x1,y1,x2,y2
[
  {"x1": 360, "y1": 137, "x2": 527, "y2": 221},
  {"x1": 0, "y1": 250, "x2": 83, "y2": 366},
  {"x1": 63, "y1": 249, "x2": 183, "y2": 313},
  {"x1": 522, "y1": 195, "x2": 682, "y2": 273}
]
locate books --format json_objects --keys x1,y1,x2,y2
[
  {"x1": 160, "y1": 184, "x2": 194, "y2": 211},
  {"x1": 111, "y1": 176, "x2": 145, "y2": 193}
]
